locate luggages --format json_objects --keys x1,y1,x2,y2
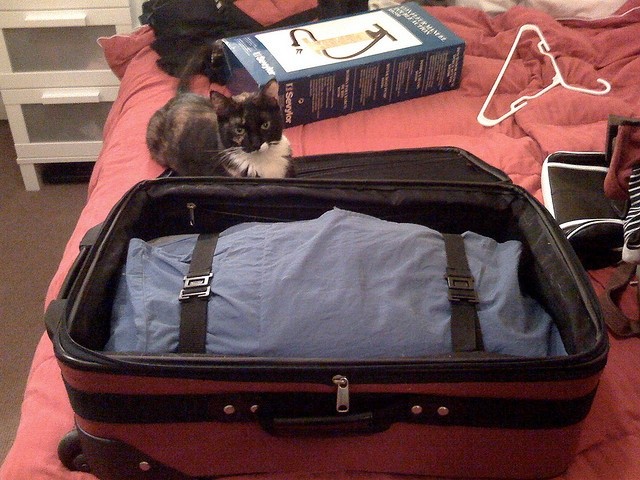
[{"x1": 44, "y1": 146, "x2": 609, "y2": 480}]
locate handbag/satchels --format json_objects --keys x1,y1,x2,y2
[{"x1": 540, "y1": 149, "x2": 628, "y2": 261}]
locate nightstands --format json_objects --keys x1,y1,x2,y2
[{"x1": 0, "y1": 1, "x2": 139, "y2": 195}]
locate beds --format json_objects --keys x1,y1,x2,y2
[{"x1": 0, "y1": 0, "x2": 640, "y2": 480}]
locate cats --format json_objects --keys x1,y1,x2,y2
[{"x1": 146, "y1": 43, "x2": 296, "y2": 178}]
